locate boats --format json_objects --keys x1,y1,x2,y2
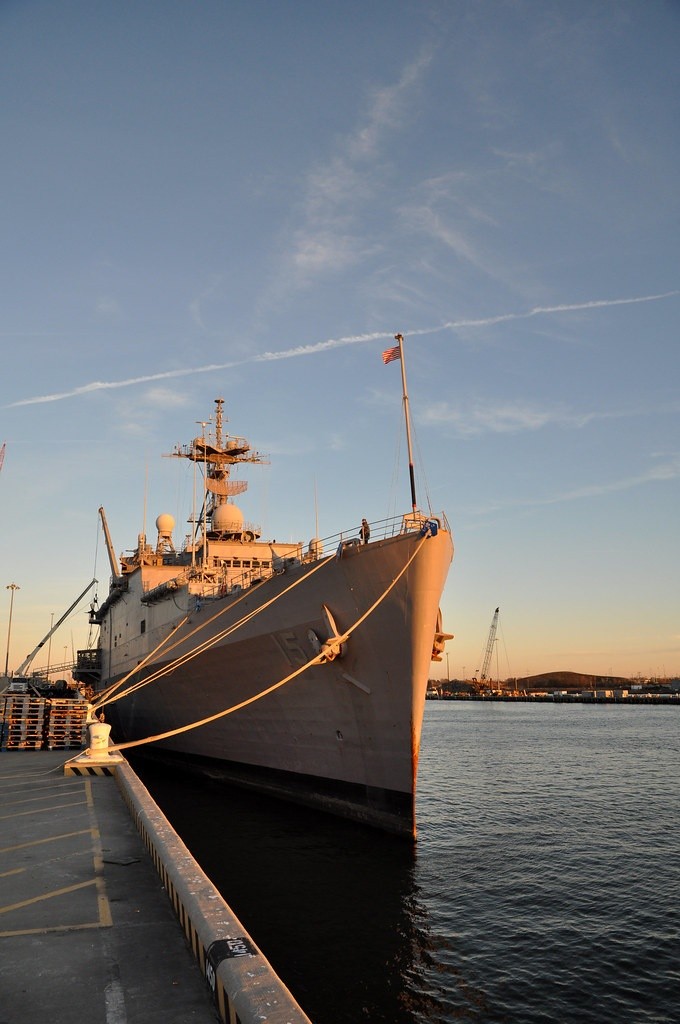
[{"x1": 70, "y1": 332, "x2": 453, "y2": 840}]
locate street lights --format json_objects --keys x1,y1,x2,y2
[{"x1": 5, "y1": 580, "x2": 21, "y2": 678}]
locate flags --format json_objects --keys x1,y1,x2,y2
[{"x1": 381, "y1": 347, "x2": 400, "y2": 365}]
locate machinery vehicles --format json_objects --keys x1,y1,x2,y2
[
  {"x1": 7, "y1": 578, "x2": 99, "y2": 694},
  {"x1": 472, "y1": 607, "x2": 500, "y2": 690}
]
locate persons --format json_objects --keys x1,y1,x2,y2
[{"x1": 359, "y1": 519, "x2": 370, "y2": 543}]
